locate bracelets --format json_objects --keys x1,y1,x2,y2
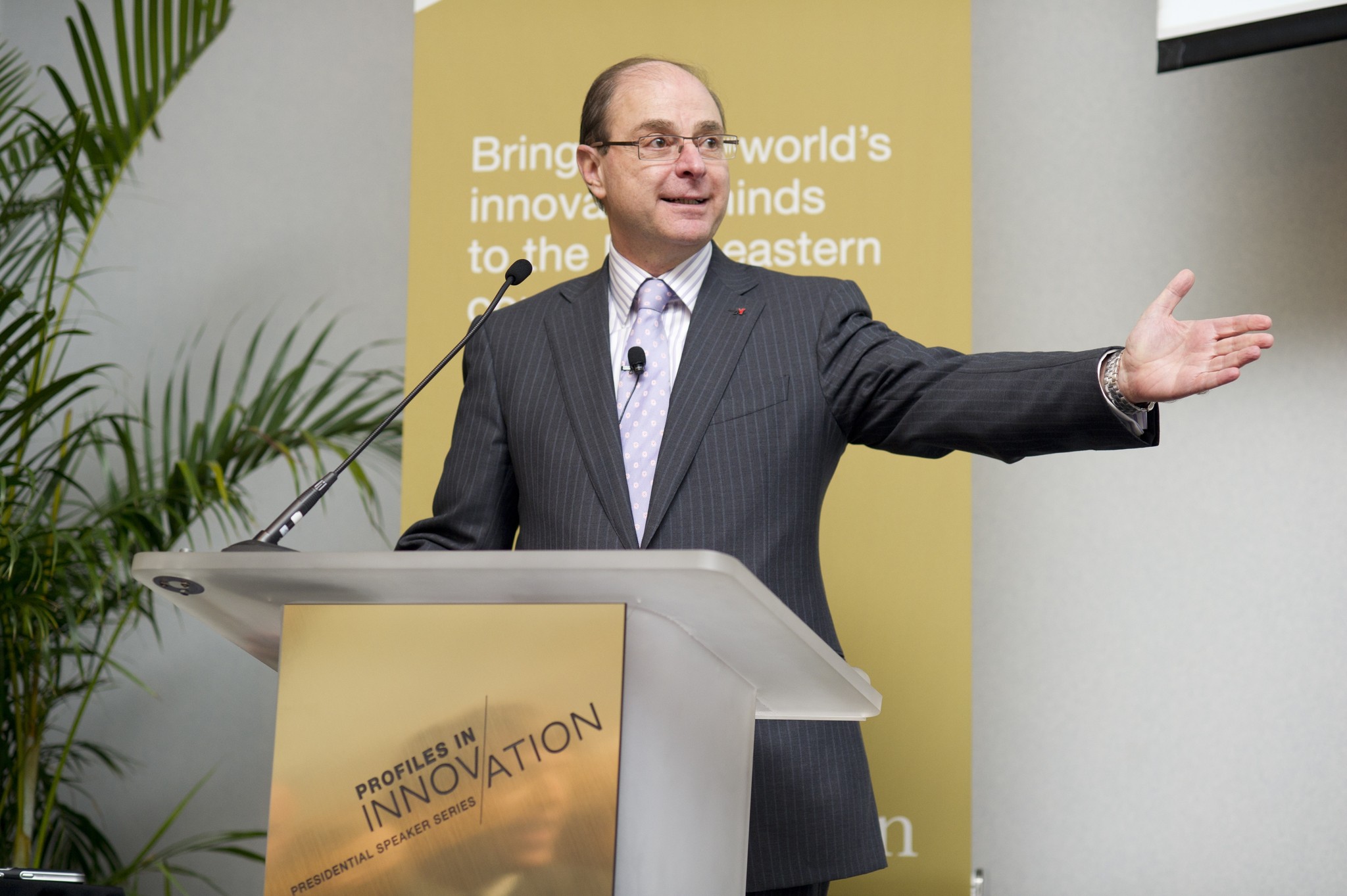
[{"x1": 1104, "y1": 349, "x2": 1156, "y2": 415}]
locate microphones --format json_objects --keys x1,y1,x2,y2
[
  {"x1": 221, "y1": 259, "x2": 532, "y2": 553},
  {"x1": 628, "y1": 346, "x2": 646, "y2": 374}
]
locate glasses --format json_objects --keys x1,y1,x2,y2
[{"x1": 590, "y1": 134, "x2": 739, "y2": 162}]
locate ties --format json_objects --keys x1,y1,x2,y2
[{"x1": 616, "y1": 279, "x2": 675, "y2": 548}]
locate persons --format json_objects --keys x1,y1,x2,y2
[{"x1": 393, "y1": 58, "x2": 1274, "y2": 896}]
